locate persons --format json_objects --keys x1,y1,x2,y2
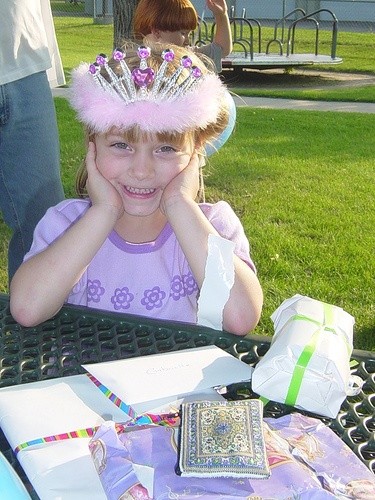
[
  {"x1": 131, "y1": 0, "x2": 234, "y2": 73},
  {"x1": 0, "y1": 0, "x2": 66, "y2": 293},
  {"x1": 9, "y1": 39, "x2": 264, "y2": 335}
]
